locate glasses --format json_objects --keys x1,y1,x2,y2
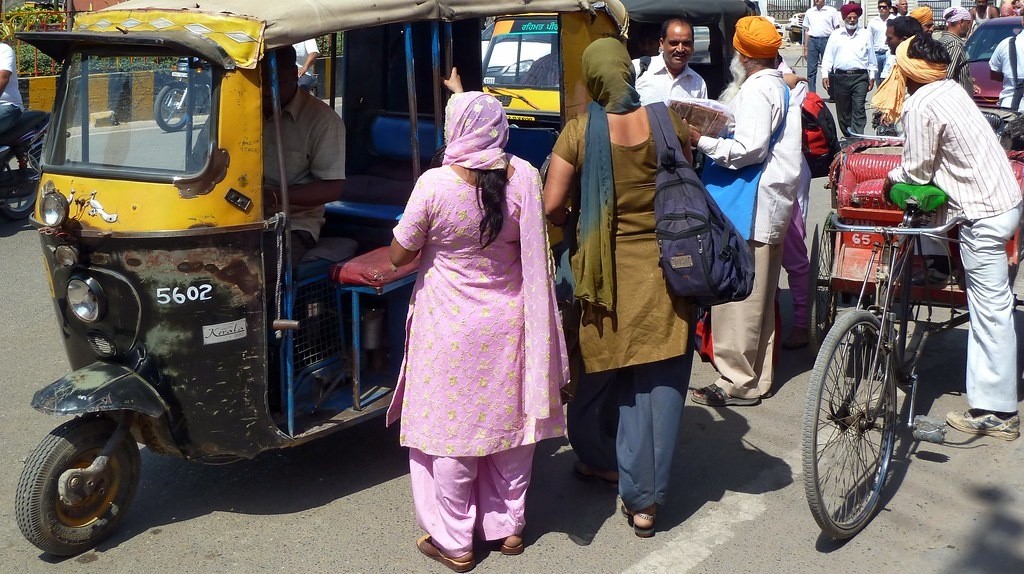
[{"x1": 878, "y1": 5, "x2": 889, "y2": 9}]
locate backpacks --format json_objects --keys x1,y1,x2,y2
[
  {"x1": 801, "y1": 92, "x2": 843, "y2": 179},
  {"x1": 646, "y1": 102, "x2": 755, "y2": 306}
]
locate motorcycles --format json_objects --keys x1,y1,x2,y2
[
  {"x1": 155, "y1": 56, "x2": 215, "y2": 133},
  {"x1": 0, "y1": 112, "x2": 71, "y2": 222}
]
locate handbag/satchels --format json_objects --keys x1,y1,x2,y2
[{"x1": 701, "y1": 77, "x2": 793, "y2": 239}]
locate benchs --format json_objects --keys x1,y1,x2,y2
[
  {"x1": 278, "y1": 237, "x2": 357, "y2": 410},
  {"x1": 318, "y1": 114, "x2": 562, "y2": 224},
  {"x1": 330, "y1": 243, "x2": 424, "y2": 407},
  {"x1": 839, "y1": 139, "x2": 1024, "y2": 213}
]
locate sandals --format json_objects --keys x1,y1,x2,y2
[
  {"x1": 621, "y1": 503, "x2": 656, "y2": 537},
  {"x1": 574, "y1": 460, "x2": 619, "y2": 483}
]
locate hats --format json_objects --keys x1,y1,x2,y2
[
  {"x1": 841, "y1": 4, "x2": 862, "y2": 19},
  {"x1": 910, "y1": 6, "x2": 933, "y2": 24},
  {"x1": 732, "y1": 16, "x2": 781, "y2": 58}
]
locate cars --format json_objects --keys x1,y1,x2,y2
[{"x1": 954, "y1": 15, "x2": 1024, "y2": 109}]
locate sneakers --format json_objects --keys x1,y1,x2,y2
[{"x1": 946, "y1": 410, "x2": 1020, "y2": 440}]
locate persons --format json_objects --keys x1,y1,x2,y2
[
  {"x1": 187, "y1": 43, "x2": 346, "y2": 302},
  {"x1": 630, "y1": 16, "x2": 708, "y2": 106},
  {"x1": 0, "y1": 42, "x2": 24, "y2": 134},
  {"x1": 880, "y1": 34, "x2": 1024, "y2": 442},
  {"x1": 292, "y1": 38, "x2": 320, "y2": 93},
  {"x1": 385, "y1": 65, "x2": 571, "y2": 573},
  {"x1": 542, "y1": 37, "x2": 698, "y2": 537},
  {"x1": 520, "y1": 47, "x2": 558, "y2": 86},
  {"x1": 683, "y1": 0, "x2": 1024, "y2": 407}
]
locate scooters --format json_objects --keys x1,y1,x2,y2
[{"x1": 14, "y1": 0, "x2": 770, "y2": 552}]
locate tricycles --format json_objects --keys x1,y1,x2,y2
[{"x1": 808, "y1": 128, "x2": 1024, "y2": 540}]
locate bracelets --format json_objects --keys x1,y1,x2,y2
[
  {"x1": 271, "y1": 191, "x2": 278, "y2": 208},
  {"x1": 551, "y1": 208, "x2": 571, "y2": 227}
]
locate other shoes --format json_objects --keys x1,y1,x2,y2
[{"x1": 782, "y1": 326, "x2": 810, "y2": 348}]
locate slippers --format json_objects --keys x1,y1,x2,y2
[
  {"x1": 691, "y1": 384, "x2": 760, "y2": 407},
  {"x1": 496, "y1": 538, "x2": 524, "y2": 556},
  {"x1": 417, "y1": 535, "x2": 475, "y2": 573}
]
